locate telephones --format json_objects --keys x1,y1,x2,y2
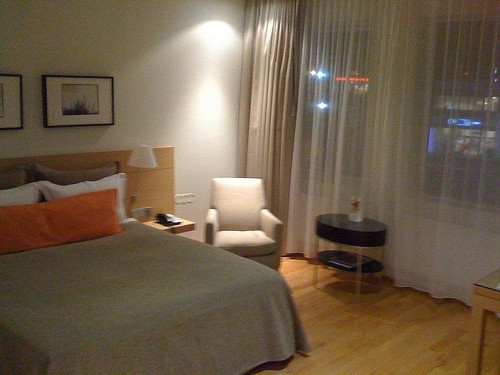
[{"x1": 156, "y1": 213, "x2": 180, "y2": 226}]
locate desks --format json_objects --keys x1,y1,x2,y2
[{"x1": 466, "y1": 269, "x2": 500, "y2": 375}]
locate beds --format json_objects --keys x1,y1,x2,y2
[{"x1": 0, "y1": 146, "x2": 311, "y2": 375}]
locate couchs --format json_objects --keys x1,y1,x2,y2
[{"x1": 203, "y1": 176, "x2": 285, "y2": 271}]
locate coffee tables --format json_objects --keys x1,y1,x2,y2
[{"x1": 312, "y1": 213, "x2": 387, "y2": 304}]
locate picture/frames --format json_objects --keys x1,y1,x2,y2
[
  {"x1": 0, "y1": 73, "x2": 24, "y2": 130},
  {"x1": 42, "y1": 72, "x2": 115, "y2": 128}
]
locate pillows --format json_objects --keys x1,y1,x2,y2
[
  {"x1": 0, "y1": 167, "x2": 38, "y2": 190},
  {"x1": 39, "y1": 172, "x2": 128, "y2": 226},
  {"x1": 29, "y1": 158, "x2": 120, "y2": 185},
  {"x1": 1, "y1": 182, "x2": 43, "y2": 207},
  {"x1": 0, "y1": 188, "x2": 125, "y2": 256}
]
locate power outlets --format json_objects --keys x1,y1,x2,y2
[
  {"x1": 131, "y1": 207, "x2": 152, "y2": 218},
  {"x1": 174, "y1": 194, "x2": 196, "y2": 204}
]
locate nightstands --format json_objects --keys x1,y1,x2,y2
[{"x1": 142, "y1": 216, "x2": 196, "y2": 234}]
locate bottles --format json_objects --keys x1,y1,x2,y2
[{"x1": 348, "y1": 195, "x2": 363, "y2": 223}]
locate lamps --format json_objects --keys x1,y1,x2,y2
[{"x1": 129, "y1": 144, "x2": 159, "y2": 203}]
